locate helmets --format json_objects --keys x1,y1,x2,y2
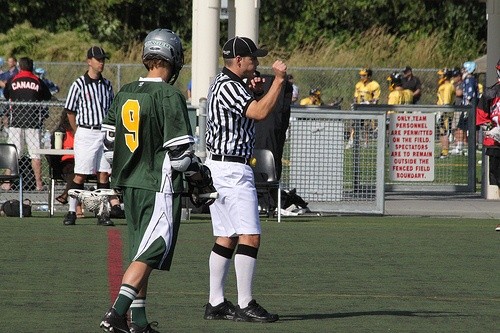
[
  {"x1": 141, "y1": 27, "x2": 184, "y2": 69},
  {"x1": 309, "y1": 88, "x2": 322, "y2": 95},
  {"x1": 437, "y1": 67, "x2": 460, "y2": 79},
  {"x1": 463, "y1": 61, "x2": 476, "y2": 74},
  {"x1": 358, "y1": 66, "x2": 373, "y2": 83},
  {"x1": 387, "y1": 71, "x2": 403, "y2": 85},
  {"x1": 496, "y1": 58, "x2": 500, "y2": 82},
  {"x1": 33, "y1": 67, "x2": 46, "y2": 80}
]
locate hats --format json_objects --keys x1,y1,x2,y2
[
  {"x1": 286, "y1": 74, "x2": 294, "y2": 80},
  {"x1": 402, "y1": 65, "x2": 412, "y2": 72},
  {"x1": 222, "y1": 36, "x2": 268, "y2": 59},
  {"x1": 86, "y1": 46, "x2": 110, "y2": 60}
]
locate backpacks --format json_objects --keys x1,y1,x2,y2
[{"x1": 0, "y1": 198, "x2": 32, "y2": 218}]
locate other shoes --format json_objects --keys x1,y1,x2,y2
[
  {"x1": 63, "y1": 211, "x2": 77, "y2": 226},
  {"x1": 96, "y1": 213, "x2": 114, "y2": 226},
  {"x1": 108, "y1": 204, "x2": 126, "y2": 219},
  {"x1": 440, "y1": 151, "x2": 451, "y2": 158},
  {"x1": 56, "y1": 192, "x2": 69, "y2": 204},
  {"x1": 448, "y1": 142, "x2": 468, "y2": 157}
]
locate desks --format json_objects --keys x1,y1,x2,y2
[{"x1": 33, "y1": 149, "x2": 114, "y2": 217}]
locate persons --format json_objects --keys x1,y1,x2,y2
[
  {"x1": 204, "y1": 37, "x2": 288, "y2": 323},
  {"x1": 385, "y1": 70, "x2": 414, "y2": 156},
  {"x1": 288, "y1": 75, "x2": 299, "y2": 105},
  {"x1": 0, "y1": 57, "x2": 52, "y2": 192},
  {"x1": 476, "y1": 59, "x2": 500, "y2": 231},
  {"x1": 62, "y1": 46, "x2": 116, "y2": 226},
  {"x1": 100, "y1": 27, "x2": 194, "y2": 333},
  {"x1": 56, "y1": 106, "x2": 121, "y2": 218},
  {"x1": 299, "y1": 87, "x2": 324, "y2": 106},
  {"x1": 0, "y1": 53, "x2": 59, "y2": 131},
  {"x1": 436, "y1": 61, "x2": 486, "y2": 159},
  {"x1": 345, "y1": 67, "x2": 382, "y2": 151},
  {"x1": 399, "y1": 66, "x2": 422, "y2": 105}
]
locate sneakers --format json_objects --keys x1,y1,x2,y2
[
  {"x1": 232, "y1": 298, "x2": 279, "y2": 323},
  {"x1": 98, "y1": 307, "x2": 132, "y2": 333},
  {"x1": 203, "y1": 297, "x2": 237, "y2": 321},
  {"x1": 126, "y1": 318, "x2": 163, "y2": 333}
]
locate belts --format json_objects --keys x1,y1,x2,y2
[
  {"x1": 79, "y1": 123, "x2": 101, "y2": 130},
  {"x1": 212, "y1": 153, "x2": 250, "y2": 166}
]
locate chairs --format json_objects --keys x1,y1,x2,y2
[
  {"x1": 0, "y1": 143, "x2": 23, "y2": 218},
  {"x1": 248, "y1": 148, "x2": 281, "y2": 224}
]
